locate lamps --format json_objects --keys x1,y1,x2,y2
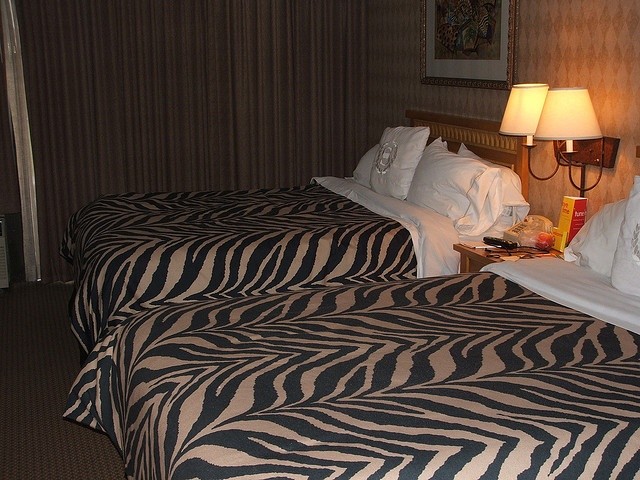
[
  {"x1": 534, "y1": 88, "x2": 604, "y2": 191},
  {"x1": 498, "y1": 84, "x2": 560, "y2": 181}
]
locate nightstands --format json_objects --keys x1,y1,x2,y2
[{"x1": 453, "y1": 239, "x2": 562, "y2": 274}]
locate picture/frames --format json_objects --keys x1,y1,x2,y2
[{"x1": 419, "y1": 0, "x2": 520, "y2": 90}]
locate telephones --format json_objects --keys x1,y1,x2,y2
[{"x1": 504, "y1": 215, "x2": 553, "y2": 243}]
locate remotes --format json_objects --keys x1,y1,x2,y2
[{"x1": 483, "y1": 236, "x2": 517, "y2": 250}]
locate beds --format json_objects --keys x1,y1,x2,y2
[
  {"x1": 59, "y1": 109, "x2": 532, "y2": 357},
  {"x1": 61, "y1": 146, "x2": 639, "y2": 480}
]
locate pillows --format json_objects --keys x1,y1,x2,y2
[
  {"x1": 352, "y1": 143, "x2": 379, "y2": 189},
  {"x1": 562, "y1": 197, "x2": 628, "y2": 278},
  {"x1": 407, "y1": 137, "x2": 501, "y2": 237},
  {"x1": 370, "y1": 126, "x2": 431, "y2": 201},
  {"x1": 612, "y1": 175, "x2": 640, "y2": 299},
  {"x1": 457, "y1": 143, "x2": 530, "y2": 234}
]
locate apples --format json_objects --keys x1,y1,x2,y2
[{"x1": 534, "y1": 232, "x2": 553, "y2": 251}]
